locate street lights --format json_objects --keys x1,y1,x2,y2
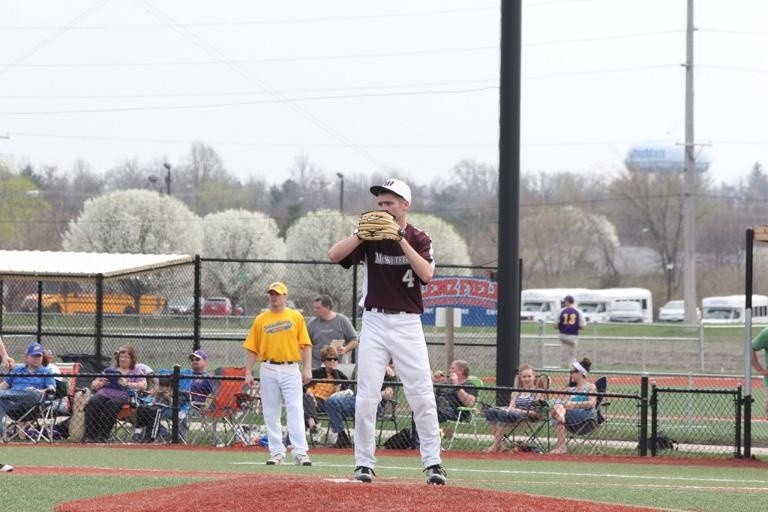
[
  {"x1": 147, "y1": 173, "x2": 159, "y2": 190},
  {"x1": 335, "y1": 171, "x2": 344, "y2": 216},
  {"x1": 162, "y1": 162, "x2": 172, "y2": 195}
]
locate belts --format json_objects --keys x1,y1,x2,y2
[
  {"x1": 366, "y1": 308, "x2": 399, "y2": 314},
  {"x1": 264, "y1": 360, "x2": 293, "y2": 364}
]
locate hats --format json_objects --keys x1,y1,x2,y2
[
  {"x1": 27, "y1": 342, "x2": 43, "y2": 356},
  {"x1": 370, "y1": 178, "x2": 412, "y2": 206},
  {"x1": 267, "y1": 282, "x2": 288, "y2": 294},
  {"x1": 189, "y1": 349, "x2": 207, "y2": 360}
]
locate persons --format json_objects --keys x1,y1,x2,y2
[
  {"x1": 555, "y1": 296, "x2": 586, "y2": 369},
  {"x1": 326, "y1": 177, "x2": 450, "y2": 484},
  {"x1": 241, "y1": 283, "x2": 394, "y2": 467},
  {"x1": 384, "y1": 357, "x2": 598, "y2": 455},
  {"x1": 751, "y1": 328, "x2": 768, "y2": 390},
  {"x1": 0, "y1": 342, "x2": 213, "y2": 472}
]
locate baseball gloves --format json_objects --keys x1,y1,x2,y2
[{"x1": 358, "y1": 210, "x2": 405, "y2": 241}]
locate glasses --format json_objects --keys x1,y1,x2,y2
[
  {"x1": 190, "y1": 358, "x2": 200, "y2": 362},
  {"x1": 569, "y1": 371, "x2": 577, "y2": 375},
  {"x1": 327, "y1": 357, "x2": 338, "y2": 361}
]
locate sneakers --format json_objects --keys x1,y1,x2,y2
[
  {"x1": 423, "y1": 464, "x2": 447, "y2": 484},
  {"x1": 294, "y1": 454, "x2": 312, "y2": 466},
  {"x1": 331, "y1": 436, "x2": 348, "y2": 447},
  {"x1": 0, "y1": 463, "x2": 13, "y2": 472},
  {"x1": 354, "y1": 467, "x2": 376, "y2": 482},
  {"x1": 266, "y1": 454, "x2": 284, "y2": 465}
]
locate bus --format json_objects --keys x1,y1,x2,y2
[
  {"x1": 521, "y1": 288, "x2": 589, "y2": 323},
  {"x1": 701, "y1": 295, "x2": 768, "y2": 324},
  {"x1": 20, "y1": 276, "x2": 169, "y2": 317},
  {"x1": 572, "y1": 286, "x2": 653, "y2": 323}
]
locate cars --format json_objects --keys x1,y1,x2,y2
[
  {"x1": 259, "y1": 301, "x2": 305, "y2": 317},
  {"x1": 658, "y1": 299, "x2": 700, "y2": 322},
  {"x1": 609, "y1": 301, "x2": 644, "y2": 322},
  {"x1": 200, "y1": 297, "x2": 245, "y2": 316},
  {"x1": 170, "y1": 295, "x2": 204, "y2": 315}
]
